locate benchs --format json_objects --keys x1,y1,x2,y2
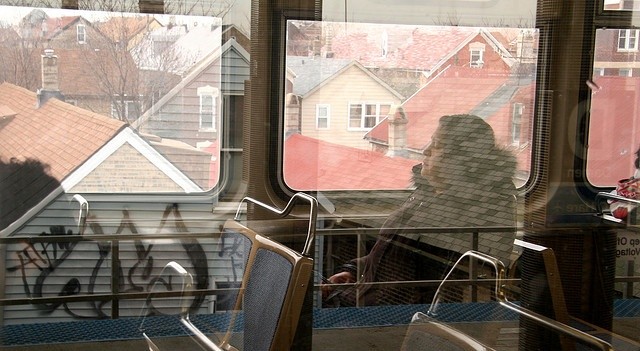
[
  {"x1": 399, "y1": 251, "x2": 609, "y2": 351},
  {"x1": 139, "y1": 192, "x2": 317, "y2": 349},
  {"x1": 514, "y1": 238, "x2": 640, "y2": 350}
]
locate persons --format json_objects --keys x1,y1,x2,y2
[
  {"x1": 609, "y1": 149, "x2": 640, "y2": 224},
  {"x1": 322, "y1": 114, "x2": 517, "y2": 307}
]
